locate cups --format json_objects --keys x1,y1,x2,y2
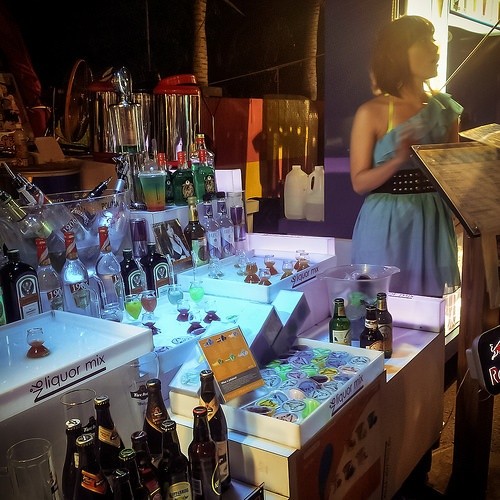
[
  {"x1": 59, "y1": 388, "x2": 97, "y2": 426},
  {"x1": 7, "y1": 438, "x2": 61, "y2": 500},
  {"x1": 209, "y1": 248, "x2": 311, "y2": 285}
]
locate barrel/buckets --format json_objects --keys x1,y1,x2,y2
[
  {"x1": 153, "y1": 74, "x2": 201, "y2": 166},
  {"x1": 82, "y1": 77, "x2": 156, "y2": 162}
]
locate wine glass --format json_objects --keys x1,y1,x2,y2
[
  {"x1": 102, "y1": 280, "x2": 220, "y2": 335},
  {"x1": 27, "y1": 327, "x2": 50, "y2": 359}
]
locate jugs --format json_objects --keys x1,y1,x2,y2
[{"x1": 317, "y1": 264, "x2": 401, "y2": 339}]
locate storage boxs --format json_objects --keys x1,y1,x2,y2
[{"x1": 170, "y1": 334, "x2": 385, "y2": 451}]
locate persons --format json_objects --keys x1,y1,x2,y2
[{"x1": 351, "y1": 15, "x2": 463, "y2": 297}]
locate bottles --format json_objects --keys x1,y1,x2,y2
[
  {"x1": 13, "y1": 123, "x2": 30, "y2": 167},
  {"x1": 199, "y1": 369, "x2": 232, "y2": 487},
  {"x1": 139, "y1": 134, "x2": 248, "y2": 268},
  {"x1": 143, "y1": 379, "x2": 173, "y2": 474},
  {"x1": 187, "y1": 406, "x2": 222, "y2": 500},
  {"x1": 131, "y1": 431, "x2": 163, "y2": 500},
  {"x1": 328, "y1": 299, "x2": 351, "y2": 347},
  {"x1": 93, "y1": 391, "x2": 125, "y2": 492},
  {"x1": 62, "y1": 418, "x2": 85, "y2": 500},
  {"x1": 0, "y1": 174, "x2": 170, "y2": 326},
  {"x1": 283, "y1": 165, "x2": 324, "y2": 224},
  {"x1": 157, "y1": 420, "x2": 193, "y2": 500},
  {"x1": 360, "y1": 304, "x2": 384, "y2": 352},
  {"x1": 119, "y1": 449, "x2": 148, "y2": 500},
  {"x1": 374, "y1": 293, "x2": 393, "y2": 357},
  {"x1": 72, "y1": 433, "x2": 115, "y2": 500}
]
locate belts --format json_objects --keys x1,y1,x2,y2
[{"x1": 371, "y1": 169, "x2": 439, "y2": 194}]
